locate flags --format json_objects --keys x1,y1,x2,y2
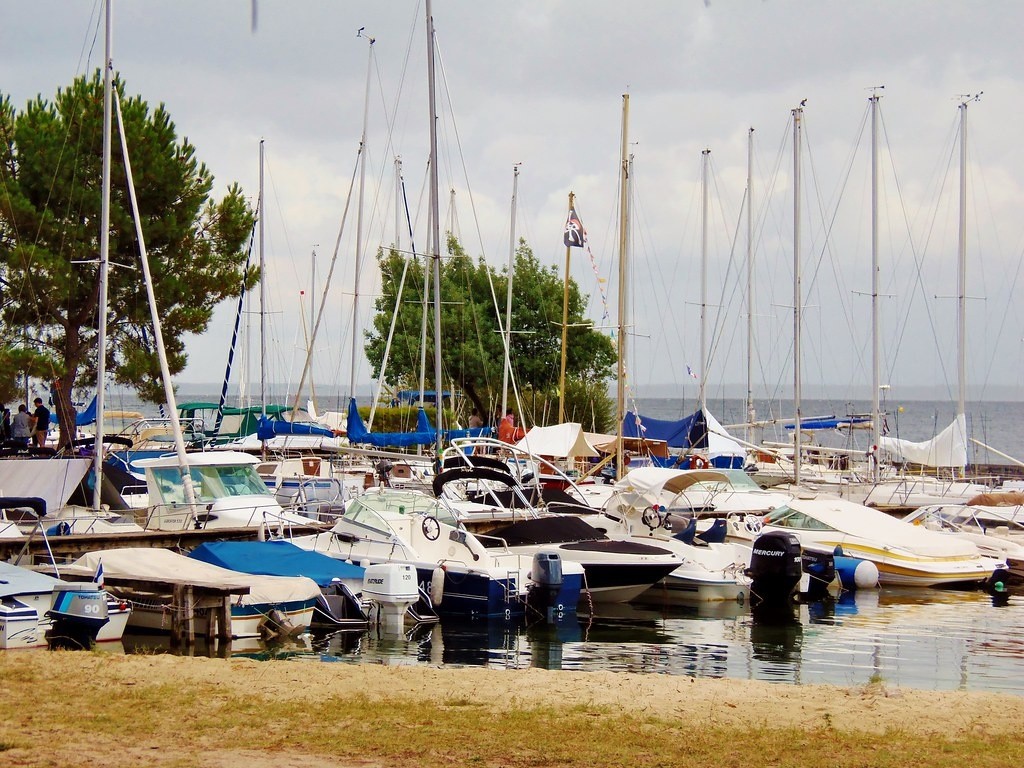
[
  {"x1": 563, "y1": 207, "x2": 584, "y2": 248},
  {"x1": 92, "y1": 560, "x2": 105, "y2": 591}
]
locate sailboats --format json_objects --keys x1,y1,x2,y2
[{"x1": 0, "y1": 0, "x2": 1024, "y2": 654}]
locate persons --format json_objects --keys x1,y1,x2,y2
[
  {"x1": 467, "y1": 404, "x2": 515, "y2": 453},
  {"x1": 32, "y1": 397, "x2": 50, "y2": 458},
  {"x1": 0, "y1": 402, "x2": 36, "y2": 460}
]
